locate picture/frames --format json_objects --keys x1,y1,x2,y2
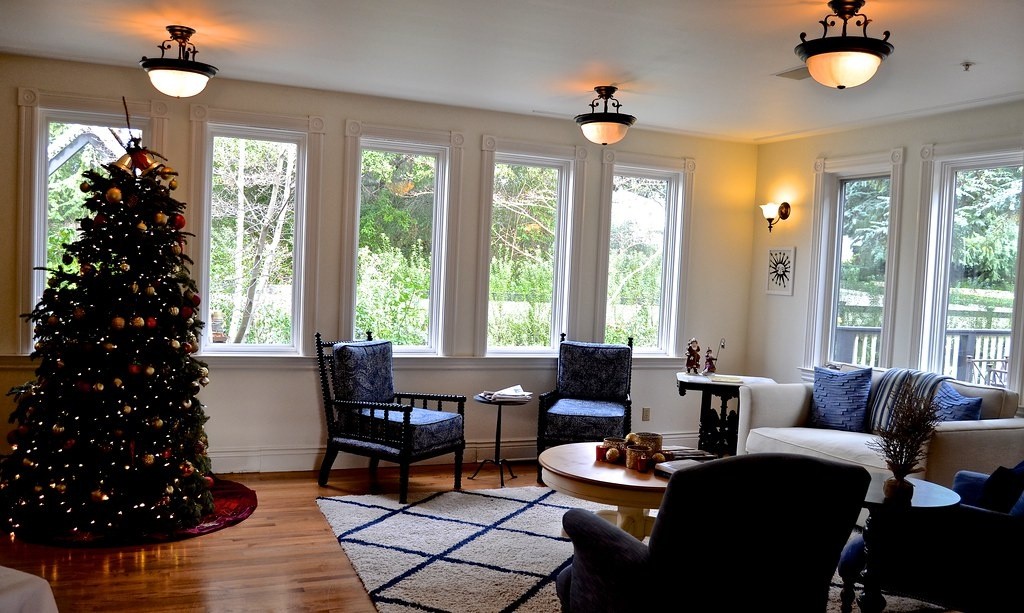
[{"x1": 765, "y1": 246, "x2": 796, "y2": 295}]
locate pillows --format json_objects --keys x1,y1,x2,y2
[
  {"x1": 928, "y1": 380, "x2": 983, "y2": 422},
  {"x1": 974, "y1": 465, "x2": 1024, "y2": 513},
  {"x1": 809, "y1": 367, "x2": 876, "y2": 431}
]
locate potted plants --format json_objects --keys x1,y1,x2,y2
[{"x1": 868, "y1": 378, "x2": 941, "y2": 506}]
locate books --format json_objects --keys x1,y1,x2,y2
[
  {"x1": 708, "y1": 375, "x2": 743, "y2": 383},
  {"x1": 654, "y1": 446, "x2": 718, "y2": 478}
]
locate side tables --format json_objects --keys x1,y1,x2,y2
[
  {"x1": 467, "y1": 392, "x2": 532, "y2": 490},
  {"x1": 677, "y1": 372, "x2": 774, "y2": 456},
  {"x1": 855, "y1": 471, "x2": 961, "y2": 613}
]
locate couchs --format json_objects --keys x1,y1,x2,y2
[
  {"x1": 556, "y1": 452, "x2": 870, "y2": 613},
  {"x1": 837, "y1": 468, "x2": 1024, "y2": 613},
  {"x1": 735, "y1": 363, "x2": 1024, "y2": 514},
  {"x1": 538, "y1": 332, "x2": 636, "y2": 489},
  {"x1": 315, "y1": 331, "x2": 467, "y2": 505}
]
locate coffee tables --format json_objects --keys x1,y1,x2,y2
[{"x1": 539, "y1": 441, "x2": 723, "y2": 542}]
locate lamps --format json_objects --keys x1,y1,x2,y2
[
  {"x1": 138, "y1": 24, "x2": 216, "y2": 99},
  {"x1": 794, "y1": 0, "x2": 894, "y2": 89},
  {"x1": 574, "y1": 82, "x2": 636, "y2": 146},
  {"x1": 758, "y1": 201, "x2": 794, "y2": 228}
]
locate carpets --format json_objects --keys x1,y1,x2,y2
[{"x1": 316, "y1": 485, "x2": 946, "y2": 613}]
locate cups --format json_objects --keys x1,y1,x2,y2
[
  {"x1": 603, "y1": 437, "x2": 629, "y2": 465},
  {"x1": 635, "y1": 431, "x2": 662, "y2": 465},
  {"x1": 626, "y1": 445, "x2": 652, "y2": 469},
  {"x1": 596, "y1": 445, "x2": 609, "y2": 462},
  {"x1": 637, "y1": 456, "x2": 650, "y2": 472}
]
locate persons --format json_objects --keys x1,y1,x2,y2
[
  {"x1": 703, "y1": 347, "x2": 716, "y2": 373},
  {"x1": 685, "y1": 338, "x2": 700, "y2": 375}
]
